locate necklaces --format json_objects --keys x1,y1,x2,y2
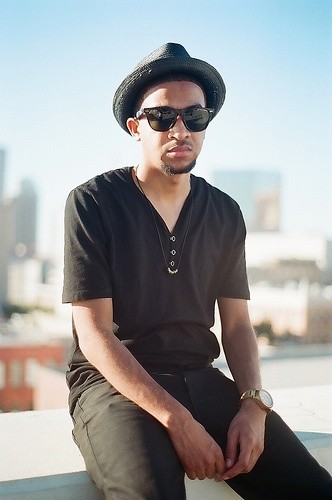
[{"x1": 136, "y1": 165, "x2": 194, "y2": 275}]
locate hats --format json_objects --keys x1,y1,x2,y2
[{"x1": 113, "y1": 42, "x2": 226, "y2": 135}]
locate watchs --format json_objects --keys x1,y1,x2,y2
[{"x1": 239, "y1": 387, "x2": 274, "y2": 416}]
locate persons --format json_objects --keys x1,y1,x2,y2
[
  {"x1": 158, "y1": 110, "x2": 163, "y2": 123},
  {"x1": 62, "y1": 43, "x2": 332, "y2": 500}
]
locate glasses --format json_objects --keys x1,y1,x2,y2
[{"x1": 135, "y1": 106, "x2": 215, "y2": 133}]
